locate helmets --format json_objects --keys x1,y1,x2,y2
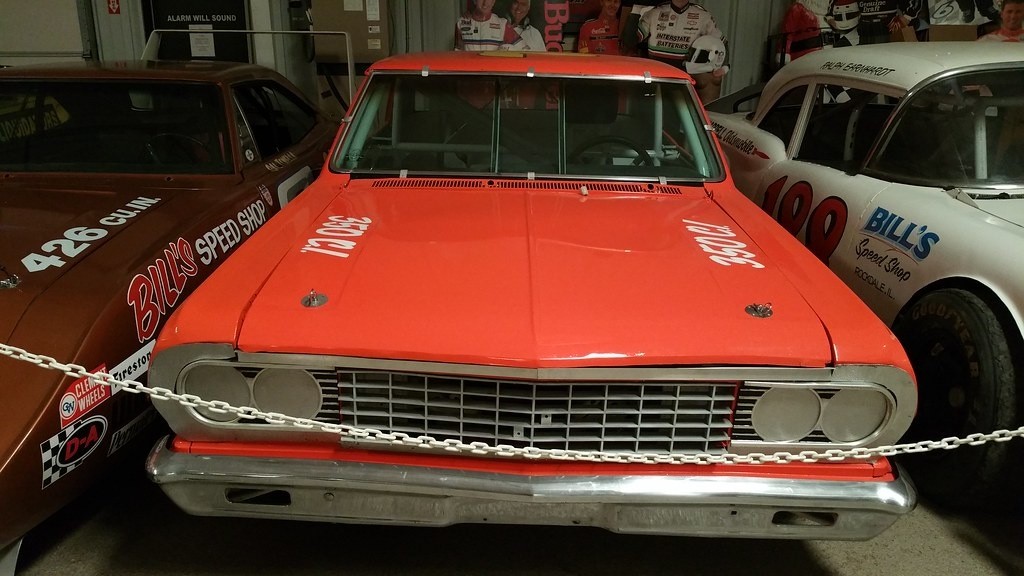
[
  {"x1": 832, "y1": 1, "x2": 861, "y2": 29},
  {"x1": 685, "y1": 35, "x2": 727, "y2": 74}
]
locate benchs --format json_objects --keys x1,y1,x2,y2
[{"x1": 380, "y1": 106, "x2": 666, "y2": 177}]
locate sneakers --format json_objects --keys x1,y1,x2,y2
[
  {"x1": 963, "y1": 8, "x2": 975, "y2": 23},
  {"x1": 980, "y1": 6, "x2": 999, "y2": 20}
]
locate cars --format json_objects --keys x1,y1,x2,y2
[
  {"x1": 146, "y1": 50, "x2": 920, "y2": 541},
  {"x1": 0, "y1": 61, "x2": 341, "y2": 554},
  {"x1": 685, "y1": 41, "x2": 1024, "y2": 505}
]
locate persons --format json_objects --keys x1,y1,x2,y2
[
  {"x1": 500, "y1": 0, "x2": 554, "y2": 110},
  {"x1": 577, "y1": 0, "x2": 635, "y2": 87},
  {"x1": 619, "y1": 0, "x2": 731, "y2": 77},
  {"x1": 974, "y1": 0, "x2": 1024, "y2": 42},
  {"x1": 824, "y1": 0, "x2": 923, "y2": 46},
  {"x1": 454, "y1": 0, "x2": 531, "y2": 110},
  {"x1": 775, "y1": 0, "x2": 833, "y2": 64}
]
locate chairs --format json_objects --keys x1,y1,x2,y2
[{"x1": 824, "y1": 105, "x2": 947, "y2": 180}]
